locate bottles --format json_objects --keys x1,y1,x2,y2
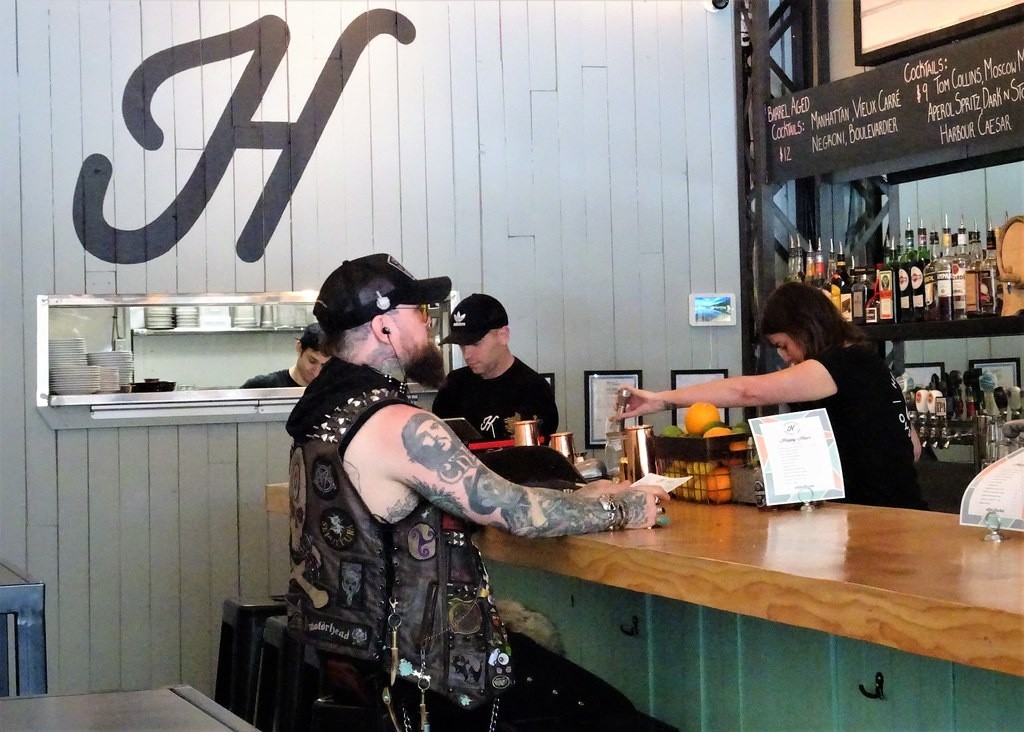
[
  {"x1": 896, "y1": 369, "x2": 1024, "y2": 470},
  {"x1": 603, "y1": 432, "x2": 624, "y2": 475},
  {"x1": 782, "y1": 210, "x2": 1009, "y2": 325},
  {"x1": 754, "y1": 459, "x2": 776, "y2": 510}
]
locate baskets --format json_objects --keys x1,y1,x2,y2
[{"x1": 649, "y1": 429, "x2": 755, "y2": 504}]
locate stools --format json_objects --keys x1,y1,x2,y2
[{"x1": 214, "y1": 596, "x2": 392, "y2": 732}]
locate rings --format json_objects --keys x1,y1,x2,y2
[
  {"x1": 656, "y1": 514, "x2": 670, "y2": 526},
  {"x1": 657, "y1": 506, "x2": 666, "y2": 514},
  {"x1": 654, "y1": 495, "x2": 661, "y2": 505}
]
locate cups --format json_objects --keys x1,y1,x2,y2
[
  {"x1": 515, "y1": 420, "x2": 538, "y2": 447},
  {"x1": 144, "y1": 305, "x2": 200, "y2": 329},
  {"x1": 624, "y1": 423, "x2": 656, "y2": 484},
  {"x1": 234, "y1": 305, "x2": 257, "y2": 328},
  {"x1": 551, "y1": 432, "x2": 575, "y2": 465},
  {"x1": 260, "y1": 305, "x2": 275, "y2": 328}
]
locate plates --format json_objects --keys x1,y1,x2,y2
[{"x1": 49, "y1": 338, "x2": 177, "y2": 394}]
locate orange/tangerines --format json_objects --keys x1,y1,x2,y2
[{"x1": 661, "y1": 402, "x2": 750, "y2": 502}]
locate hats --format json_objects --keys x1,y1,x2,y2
[
  {"x1": 438, "y1": 292, "x2": 509, "y2": 346},
  {"x1": 312, "y1": 252, "x2": 452, "y2": 335}
]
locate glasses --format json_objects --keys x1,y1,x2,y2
[{"x1": 390, "y1": 303, "x2": 429, "y2": 323}]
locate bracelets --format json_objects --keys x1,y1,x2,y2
[{"x1": 597, "y1": 492, "x2": 630, "y2": 532}]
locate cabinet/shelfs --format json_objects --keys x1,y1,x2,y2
[{"x1": 733, "y1": 0, "x2": 1024, "y2": 475}]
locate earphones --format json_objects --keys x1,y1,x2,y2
[{"x1": 381, "y1": 327, "x2": 390, "y2": 334}]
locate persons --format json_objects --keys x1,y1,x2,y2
[
  {"x1": 432, "y1": 290, "x2": 559, "y2": 454},
  {"x1": 605, "y1": 281, "x2": 933, "y2": 512},
  {"x1": 288, "y1": 254, "x2": 641, "y2": 732},
  {"x1": 241, "y1": 323, "x2": 333, "y2": 390}
]
